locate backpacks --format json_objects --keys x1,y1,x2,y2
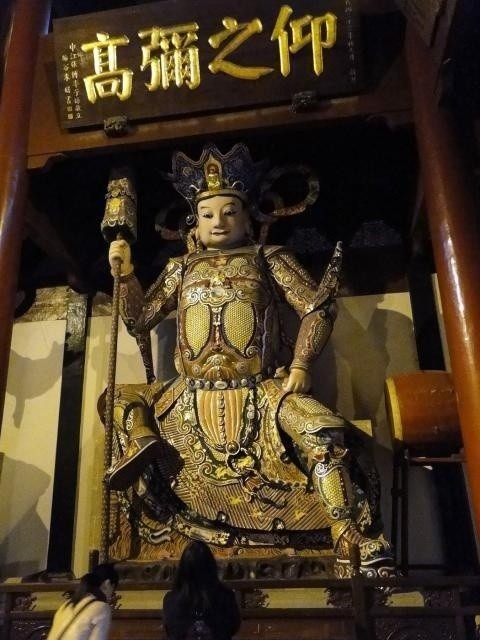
[{"x1": 177, "y1": 583, "x2": 232, "y2": 639}]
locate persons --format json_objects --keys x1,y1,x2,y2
[
  {"x1": 98, "y1": 191, "x2": 397, "y2": 565},
  {"x1": 47, "y1": 565, "x2": 119, "y2": 640},
  {"x1": 162, "y1": 541, "x2": 242, "y2": 639}
]
